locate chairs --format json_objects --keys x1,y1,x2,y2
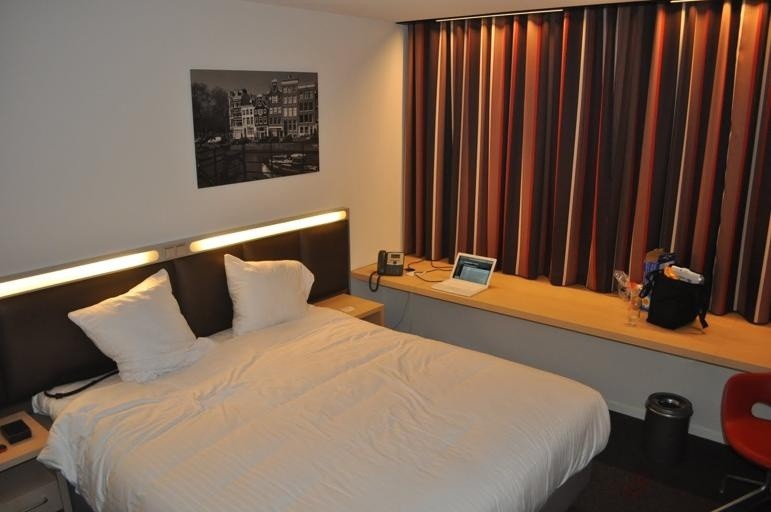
[{"x1": 707, "y1": 369, "x2": 771, "y2": 512}]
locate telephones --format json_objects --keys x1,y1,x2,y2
[{"x1": 376, "y1": 249, "x2": 405, "y2": 276}]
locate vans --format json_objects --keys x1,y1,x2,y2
[{"x1": 207, "y1": 136, "x2": 221, "y2": 145}]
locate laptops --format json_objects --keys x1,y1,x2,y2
[{"x1": 431, "y1": 251, "x2": 497, "y2": 299}]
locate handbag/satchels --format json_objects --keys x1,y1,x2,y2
[{"x1": 640, "y1": 266, "x2": 712, "y2": 331}]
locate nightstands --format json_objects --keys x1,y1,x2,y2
[
  {"x1": 1, "y1": 409, "x2": 76, "y2": 512},
  {"x1": 309, "y1": 291, "x2": 387, "y2": 329}
]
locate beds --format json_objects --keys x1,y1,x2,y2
[{"x1": 1, "y1": 207, "x2": 614, "y2": 512}]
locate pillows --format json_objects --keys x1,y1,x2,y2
[
  {"x1": 223, "y1": 252, "x2": 318, "y2": 337},
  {"x1": 67, "y1": 267, "x2": 214, "y2": 386}
]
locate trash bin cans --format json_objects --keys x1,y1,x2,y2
[{"x1": 636, "y1": 390, "x2": 695, "y2": 467}]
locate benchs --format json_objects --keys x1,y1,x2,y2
[{"x1": 349, "y1": 251, "x2": 771, "y2": 447}]
